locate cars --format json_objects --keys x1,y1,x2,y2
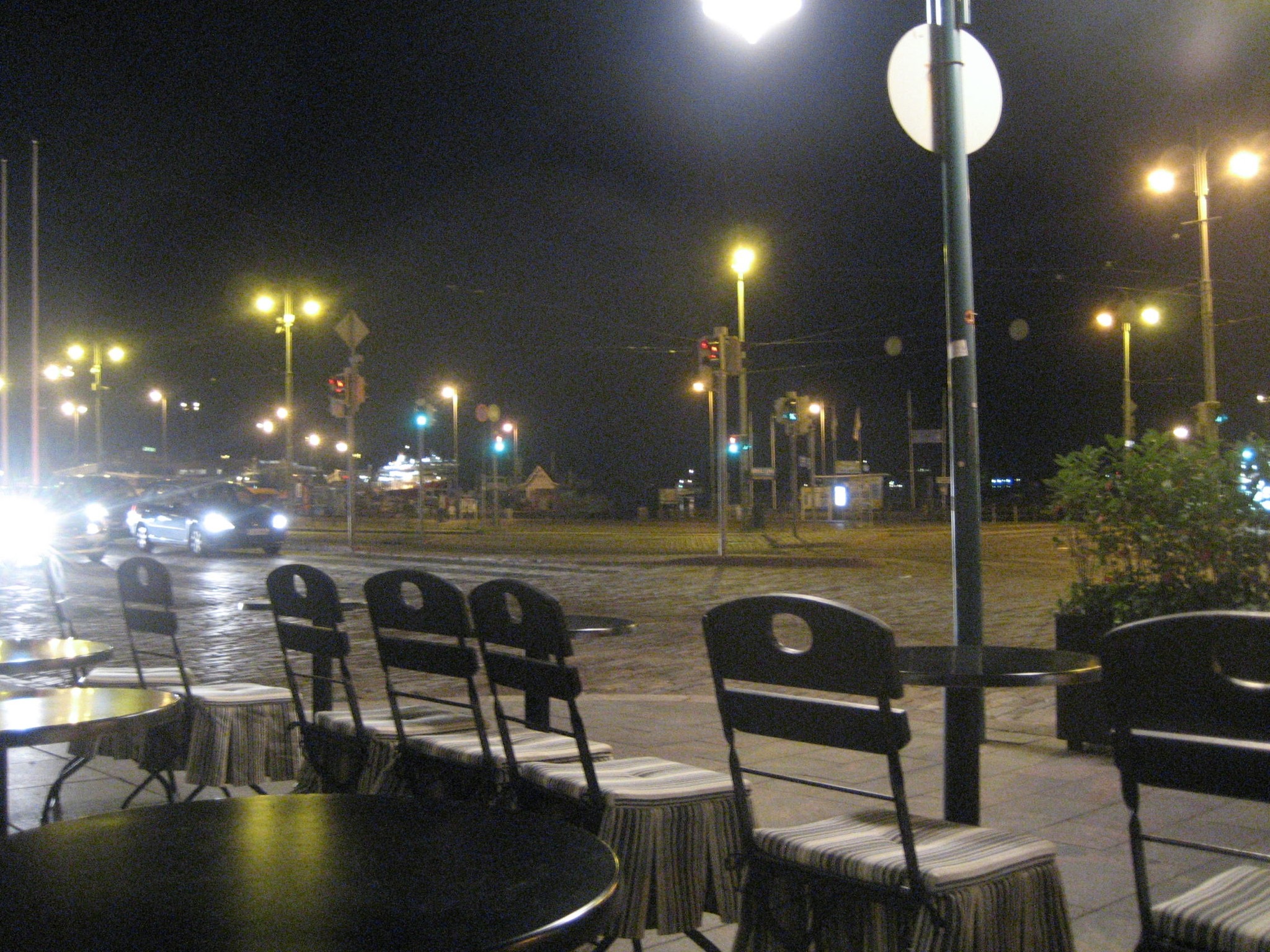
[
  {"x1": 127, "y1": 478, "x2": 290, "y2": 558},
  {"x1": 0, "y1": 485, "x2": 110, "y2": 564}
]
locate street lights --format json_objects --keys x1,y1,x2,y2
[
  {"x1": 440, "y1": 386, "x2": 464, "y2": 502},
  {"x1": 148, "y1": 389, "x2": 168, "y2": 476},
  {"x1": 65, "y1": 338, "x2": 126, "y2": 474},
  {"x1": 502, "y1": 421, "x2": 519, "y2": 485},
  {"x1": 728, "y1": 242, "x2": 759, "y2": 529},
  {"x1": 1144, "y1": 114, "x2": 1265, "y2": 458},
  {"x1": 1092, "y1": 303, "x2": 1164, "y2": 450},
  {"x1": 251, "y1": 280, "x2": 325, "y2": 514}
]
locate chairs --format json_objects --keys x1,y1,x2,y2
[
  {"x1": 1100, "y1": 610, "x2": 1269, "y2": 952},
  {"x1": 701, "y1": 593, "x2": 1074, "y2": 951},
  {"x1": 268, "y1": 563, "x2": 755, "y2": 952},
  {"x1": 43, "y1": 554, "x2": 303, "y2": 822}
]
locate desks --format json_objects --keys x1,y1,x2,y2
[
  {"x1": 524, "y1": 615, "x2": 635, "y2": 726},
  {"x1": 1, "y1": 794, "x2": 618, "y2": 952},
  {"x1": 0, "y1": 636, "x2": 115, "y2": 685},
  {"x1": 891, "y1": 644, "x2": 1098, "y2": 825},
  {"x1": 0, "y1": 687, "x2": 181, "y2": 824},
  {"x1": 241, "y1": 597, "x2": 367, "y2": 712}
]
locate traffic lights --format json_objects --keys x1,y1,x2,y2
[
  {"x1": 415, "y1": 398, "x2": 428, "y2": 429},
  {"x1": 728, "y1": 435, "x2": 743, "y2": 454},
  {"x1": 334, "y1": 373, "x2": 350, "y2": 402},
  {"x1": 707, "y1": 335, "x2": 724, "y2": 377},
  {"x1": 697, "y1": 338, "x2": 711, "y2": 378},
  {"x1": 784, "y1": 397, "x2": 798, "y2": 428},
  {"x1": 327, "y1": 375, "x2": 336, "y2": 397},
  {"x1": 771, "y1": 397, "x2": 786, "y2": 426},
  {"x1": 797, "y1": 396, "x2": 812, "y2": 435}
]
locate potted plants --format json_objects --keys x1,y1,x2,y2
[{"x1": 1045, "y1": 431, "x2": 1269, "y2": 755}]
